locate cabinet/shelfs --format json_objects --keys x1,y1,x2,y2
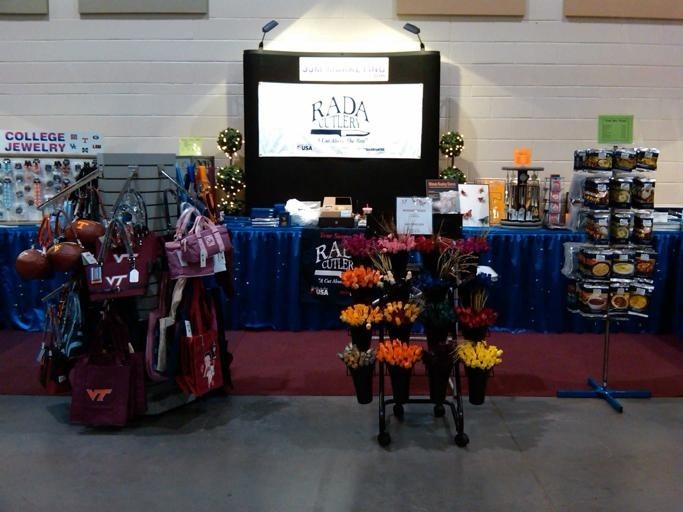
[
  {"x1": 0, "y1": 153, "x2": 98, "y2": 225},
  {"x1": 556, "y1": 147, "x2": 656, "y2": 413},
  {"x1": 0, "y1": 216, "x2": 683, "y2": 333},
  {"x1": 37, "y1": 153, "x2": 209, "y2": 416},
  {"x1": 378, "y1": 263, "x2": 470, "y2": 448}
]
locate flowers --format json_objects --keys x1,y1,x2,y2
[
  {"x1": 376, "y1": 230, "x2": 417, "y2": 254},
  {"x1": 375, "y1": 302, "x2": 421, "y2": 326},
  {"x1": 376, "y1": 339, "x2": 424, "y2": 369},
  {"x1": 377, "y1": 271, "x2": 418, "y2": 290},
  {"x1": 458, "y1": 341, "x2": 504, "y2": 371},
  {"x1": 341, "y1": 231, "x2": 376, "y2": 256},
  {"x1": 342, "y1": 264, "x2": 382, "y2": 290},
  {"x1": 462, "y1": 272, "x2": 494, "y2": 291},
  {"x1": 340, "y1": 303, "x2": 376, "y2": 330},
  {"x1": 415, "y1": 235, "x2": 443, "y2": 253},
  {"x1": 420, "y1": 249, "x2": 479, "y2": 285},
  {"x1": 441, "y1": 237, "x2": 489, "y2": 254},
  {"x1": 455, "y1": 291, "x2": 497, "y2": 328},
  {"x1": 336, "y1": 340, "x2": 378, "y2": 370}
]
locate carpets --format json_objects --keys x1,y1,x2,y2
[{"x1": 0, "y1": 332, "x2": 683, "y2": 396}]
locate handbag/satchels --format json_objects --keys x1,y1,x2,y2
[
  {"x1": 145, "y1": 271, "x2": 224, "y2": 399},
  {"x1": 38, "y1": 288, "x2": 82, "y2": 396},
  {"x1": 14, "y1": 184, "x2": 106, "y2": 280},
  {"x1": 181, "y1": 213, "x2": 232, "y2": 263},
  {"x1": 166, "y1": 208, "x2": 214, "y2": 279},
  {"x1": 67, "y1": 313, "x2": 146, "y2": 427},
  {"x1": 84, "y1": 186, "x2": 156, "y2": 301},
  {"x1": 180, "y1": 203, "x2": 227, "y2": 273}
]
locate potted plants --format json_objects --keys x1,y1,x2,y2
[
  {"x1": 424, "y1": 304, "x2": 455, "y2": 352},
  {"x1": 423, "y1": 344, "x2": 453, "y2": 404}
]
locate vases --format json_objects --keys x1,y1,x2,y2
[
  {"x1": 350, "y1": 289, "x2": 373, "y2": 303},
  {"x1": 461, "y1": 292, "x2": 481, "y2": 307},
  {"x1": 390, "y1": 368, "x2": 412, "y2": 403},
  {"x1": 392, "y1": 253, "x2": 409, "y2": 269},
  {"x1": 351, "y1": 329, "x2": 371, "y2": 347},
  {"x1": 391, "y1": 296, "x2": 409, "y2": 302},
  {"x1": 427, "y1": 255, "x2": 443, "y2": 277},
  {"x1": 473, "y1": 253, "x2": 482, "y2": 263},
  {"x1": 469, "y1": 371, "x2": 490, "y2": 405},
  {"x1": 349, "y1": 368, "x2": 372, "y2": 404},
  {"x1": 425, "y1": 288, "x2": 449, "y2": 303},
  {"x1": 351, "y1": 256, "x2": 373, "y2": 267},
  {"x1": 390, "y1": 326, "x2": 411, "y2": 341},
  {"x1": 465, "y1": 330, "x2": 489, "y2": 340}
]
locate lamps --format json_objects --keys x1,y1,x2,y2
[
  {"x1": 404, "y1": 23, "x2": 424, "y2": 50},
  {"x1": 259, "y1": 20, "x2": 279, "y2": 50}
]
